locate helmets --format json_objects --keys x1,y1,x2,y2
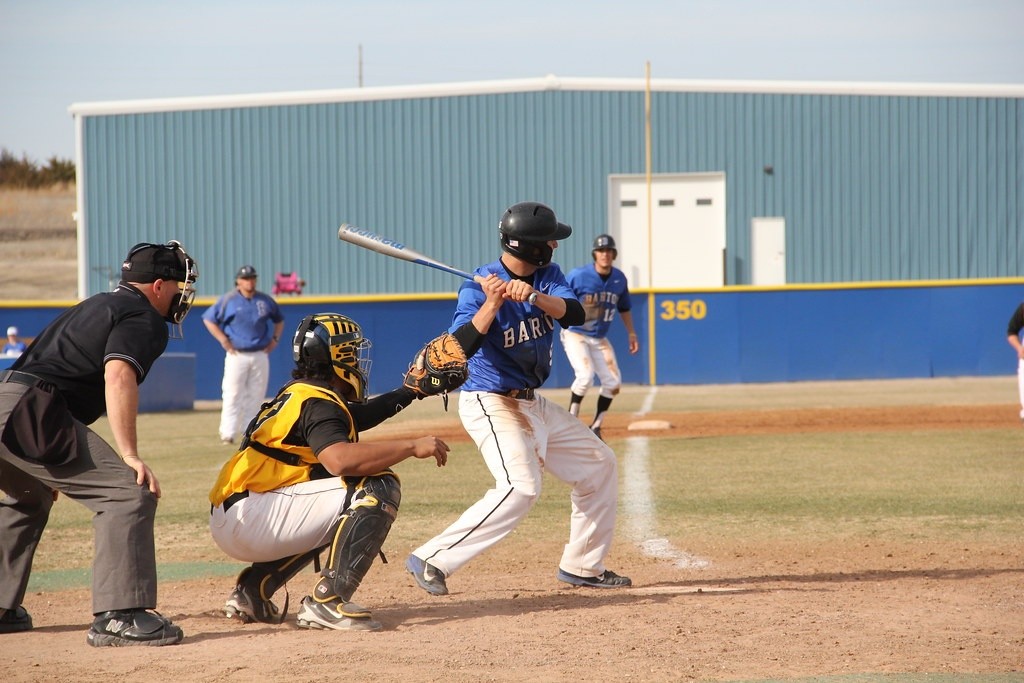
[
  {"x1": 499, "y1": 201, "x2": 572, "y2": 266},
  {"x1": 292, "y1": 313, "x2": 372, "y2": 405},
  {"x1": 592, "y1": 235, "x2": 617, "y2": 261},
  {"x1": 238, "y1": 265, "x2": 257, "y2": 278}
]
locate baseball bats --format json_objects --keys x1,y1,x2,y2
[{"x1": 338, "y1": 222, "x2": 538, "y2": 305}]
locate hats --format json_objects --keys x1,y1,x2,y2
[{"x1": 121, "y1": 244, "x2": 194, "y2": 283}]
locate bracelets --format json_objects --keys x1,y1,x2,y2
[{"x1": 273, "y1": 335, "x2": 279, "y2": 342}]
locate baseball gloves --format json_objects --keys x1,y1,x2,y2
[{"x1": 403, "y1": 335, "x2": 470, "y2": 396}]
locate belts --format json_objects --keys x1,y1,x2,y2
[
  {"x1": 491, "y1": 388, "x2": 535, "y2": 400},
  {"x1": 210, "y1": 490, "x2": 248, "y2": 515},
  {"x1": 0, "y1": 370, "x2": 38, "y2": 385}
]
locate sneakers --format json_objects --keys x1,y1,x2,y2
[
  {"x1": 296, "y1": 596, "x2": 382, "y2": 632},
  {"x1": 405, "y1": 554, "x2": 448, "y2": 596},
  {"x1": 0, "y1": 607, "x2": 33, "y2": 632},
  {"x1": 87, "y1": 610, "x2": 183, "y2": 647},
  {"x1": 223, "y1": 591, "x2": 280, "y2": 624},
  {"x1": 558, "y1": 568, "x2": 631, "y2": 588}
]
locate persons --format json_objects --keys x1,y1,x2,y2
[
  {"x1": 208, "y1": 313, "x2": 469, "y2": 632},
  {"x1": 201, "y1": 265, "x2": 285, "y2": 444},
  {"x1": 1007, "y1": 302, "x2": 1024, "y2": 419},
  {"x1": 2, "y1": 326, "x2": 26, "y2": 357},
  {"x1": 0, "y1": 239, "x2": 200, "y2": 648},
  {"x1": 560, "y1": 234, "x2": 639, "y2": 444},
  {"x1": 404, "y1": 202, "x2": 632, "y2": 596}
]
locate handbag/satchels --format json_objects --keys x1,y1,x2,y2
[{"x1": 2, "y1": 381, "x2": 80, "y2": 466}]
